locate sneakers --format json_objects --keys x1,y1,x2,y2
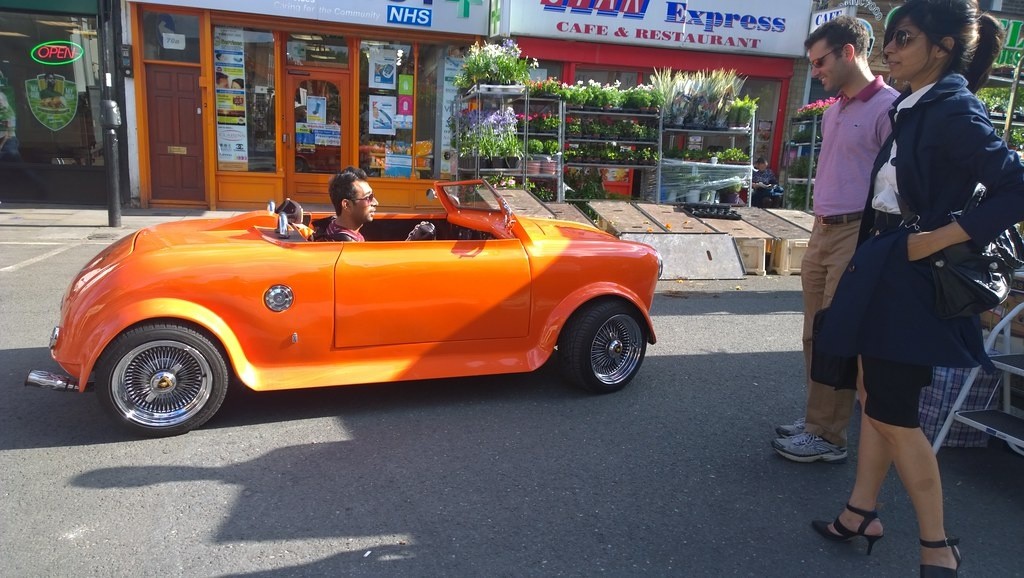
[
  {"x1": 771, "y1": 432, "x2": 848, "y2": 464},
  {"x1": 775, "y1": 418, "x2": 808, "y2": 439}
]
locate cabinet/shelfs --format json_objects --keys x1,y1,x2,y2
[
  {"x1": 655, "y1": 98, "x2": 758, "y2": 204},
  {"x1": 456, "y1": 91, "x2": 530, "y2": 187},
  {"x1": 786, "y1": 113, "x2": 829, "y2": 213},
  {"x1": 559, "y1": 110, "x2": 663, "y2": 201},
  {"x1": 519, "y1": 84, "x2": 562, "y2": 203}
]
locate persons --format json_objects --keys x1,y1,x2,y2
[
  {"x1": 753, "y1": 155, "x2": 776, "y2": 209},
  {"x1": 216, "y1": 53, "x2": 224, "y2": 61},
  {"x1": 811, "y1": 0, "x2": 1024, "y2": 578},
  {"x1": 0, "y1": 92, "x2": 23, "y2": 161},
  {"x1": 306, "y1": 165, "x2": 429, "y2": 242},
  {"x1": 39, "y1": 72, "x2": 62, "y2": 100},
  {"x1": 216, "y1": 71, "x2": 244, "y2": 90},
  {"x1": 770, "y1": 15, "x2": 901, "y2": 463}
]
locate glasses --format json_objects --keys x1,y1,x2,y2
[
  {"x1": 810, "y1": 44, "x2": 847, "y2": 68},
  {"x1": 891, "y1": 30, "x2": 942, "y2": 48},
  {"x1": 350, "y1": 193, "x2": 374, "y2": 202}
]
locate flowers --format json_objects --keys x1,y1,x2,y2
[
  {"x1": 794, "y1": 96, "x2": 841, "y2": 115},
  {"x1": 454, "y1": 39, "x2": 654, "y2": 94},
  {"x1": 478, "y1": 177, "x2": 553, "y2": 204},
  {"x1": 451, "y1": 109, "x2": 559, "y2": 156}
]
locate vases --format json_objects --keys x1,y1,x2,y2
[
  {"x1": 457, "y1": 122, "x2": 560, "y2": 174},
  {"x1": 462, "y1": 77, "x2": 655, "y2": 115},
  {"x1": 790, "y1": 111, "x2": 827, "y2": 121}
]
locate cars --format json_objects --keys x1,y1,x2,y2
[
  {"x1": 293, "y1": 144, "x2": 386, "y2": 177},
  {"x1": 26, "y1": 179, "x2": 664, "y2": 439}
]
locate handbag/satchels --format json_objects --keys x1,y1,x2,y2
[{"x1": 931, "y1": 182, "x2": 1024, "y2": 320}]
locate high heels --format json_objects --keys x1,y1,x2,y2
[
  {"x1": 920, "y1": 536, "x2": 963, "y2": 578},
  {"x1": 811, "y1": 503, "x2": 885, "y2": 556}
]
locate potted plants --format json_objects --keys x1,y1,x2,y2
[
  {"x1": 564, "y1": 110, "x2": 749, "y2": 204},
  {"x1": 788, "y1": 129, "x2": 823, "y2": 209}
]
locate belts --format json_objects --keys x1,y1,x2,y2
[{"x1": 820, "y1": 212, "x2": 862, "y2": 225}]
